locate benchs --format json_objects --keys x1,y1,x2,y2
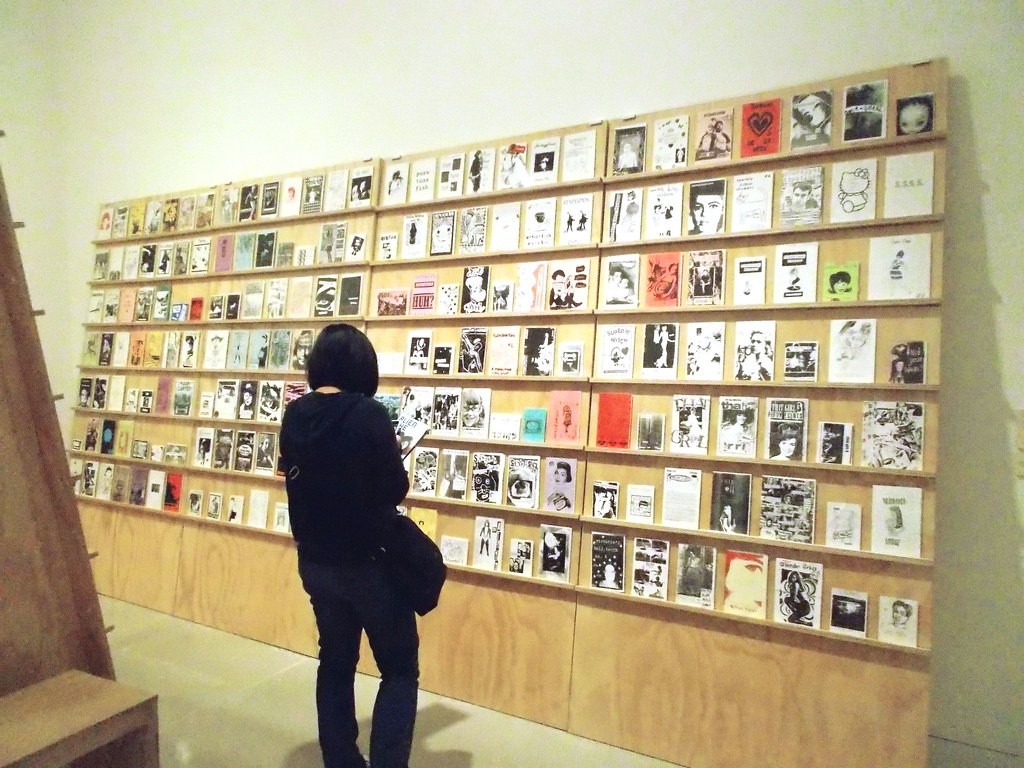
[{"x1": 0, "y1": 669, "x2": 161, "y2": 768}]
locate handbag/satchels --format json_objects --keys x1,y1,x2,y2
[{"x1": 374, "y1": 516, "x2": 447, "y2": 616}]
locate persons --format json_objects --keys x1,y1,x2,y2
[
  {"x1": 278, "y1": 323, "x2": 422, "y2": 768},
  {"x1": 69, "y1": 88, "x2": 937, "y2": 648}
]
